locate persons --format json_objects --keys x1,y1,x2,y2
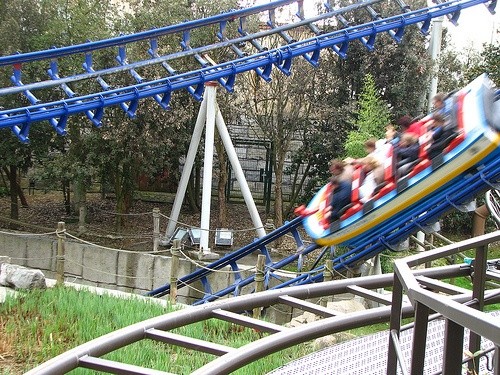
[{"x1": 325, "y1": 93, "x2": 453, "y2": 223}]
